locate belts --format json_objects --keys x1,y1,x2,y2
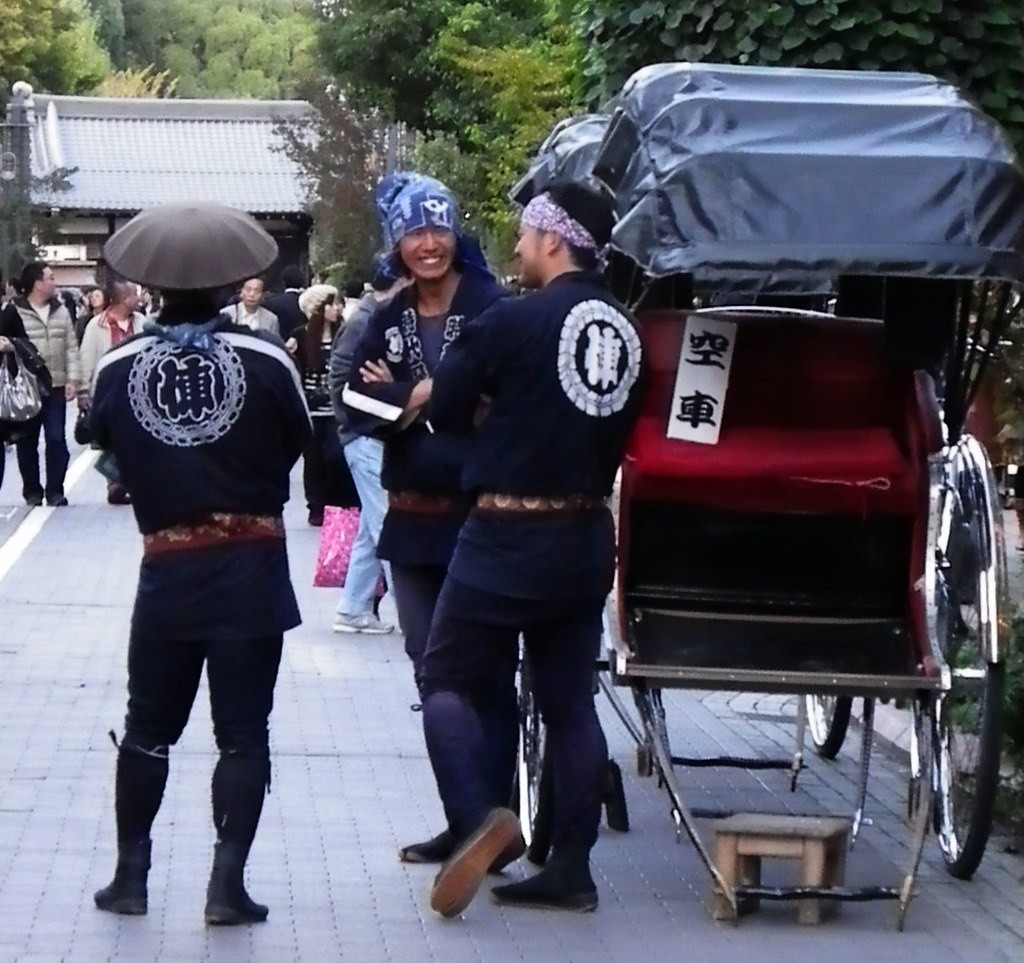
[
  {"x1": 144, "y1": 512, "x2": 286, "y2": 556},
  {"x1": 476, "y1": 492, "x2": 610, "y2": 514},
  {"x1": 388, "y1": 491, "x2": 476, "y2": 515}
]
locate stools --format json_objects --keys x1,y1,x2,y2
[{"x1": 712, "y1": 812, "x2": 852, "y2": 926}]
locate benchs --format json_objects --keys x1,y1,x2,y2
[{"x1": 618, "y1": 301, "x2": 930, "y2": 645}]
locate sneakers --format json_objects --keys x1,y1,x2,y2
[{"x1": 332, "y1": 612, "x2": 395, "y2": 634}]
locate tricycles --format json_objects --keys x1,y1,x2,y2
[{"x1": 514, "y1": 61, "x2": 1024, "y2": 880}]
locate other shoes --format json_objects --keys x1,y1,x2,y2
[
  {"x1": 401, "y1": 828, "x2": 526, "y2": 871},
  {"x1": 45, "y1": 491, "x2": 69, "y2": 507},
  {"x1": 490, "y1": 871, "x2": 600, "y2": 913},
  {"x1": 432, "y1": 808, "x2": 518, "y2": 919},
  {"x1": 308, "y1": 512, "x2": 324, "y2": 526},
  {"x1": 108, "y1": 481, "x2": 131, "y2": 504},
  {"x1": 26, "y1": 496, "x2": 42, "y2": 507}
]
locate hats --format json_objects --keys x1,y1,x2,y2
[
  {"x1": 298, "y1": 283, "x2": 338, "y2": 319},
  {"x1": 102, "y1": 200, "x2": 279, "y2": 290},
  {"x1": 283, "y1": 266, "x2": 305, "y2": 289},
  {"x1": 345, "y1": 280, "x2": 364, "y2": 299}
]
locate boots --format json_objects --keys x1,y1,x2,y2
[
  {"x1": 93, "y1": 840, "x2": 153, "y2": 914},
  {"x1": 205, "y1": 841, "x2": 268, "y2": 924},
  {"x1": 1013, "y1": 498, "x2": 1024, "y2": 550}
]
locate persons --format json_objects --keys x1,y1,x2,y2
[
  {"x1": 86, "y1": 201, "x2": 312, "y2": 926},
  {"x1": 337, "y1": 170, "x2": 511, "y2": 861},
  {"x1": 328, "y1": 252, "x2": 416, "y2": 634},
  {"x1": 220, "y1": 263, "x2": 388, "y2": 621},
  {"x1": 416, "y1": 178, "x2": 653, "y2": 920},
  {"x1": 0, "y1": 261, "x2": 163, "y2": 506}
]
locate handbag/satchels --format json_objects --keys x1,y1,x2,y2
[
  {"x1": 74, "y1": 405, "x2": 95, "y2": 445},
  {"x1": 0, "y1": 346, "x2": 42, "y2": 422},
  {"x1": 313, "y1": 488, "x2": 385, "y2": 596}
]
